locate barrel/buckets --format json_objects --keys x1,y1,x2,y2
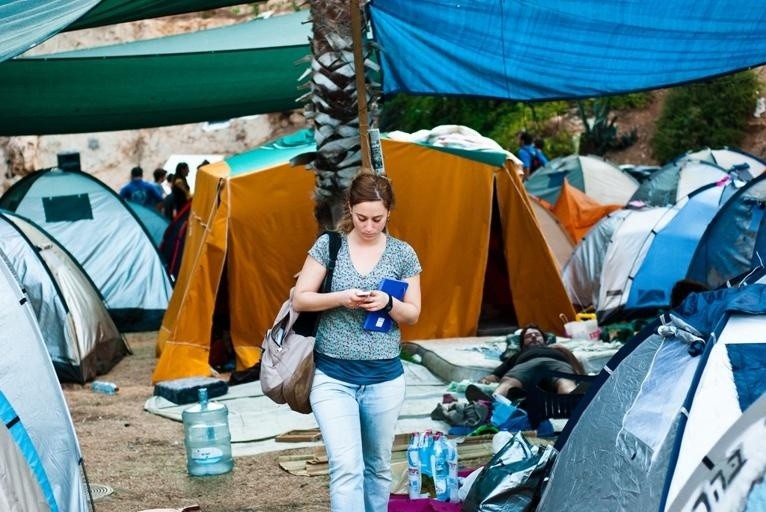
[{"x1": 182, "y1": 388, "x2": 235, "y2": 476}]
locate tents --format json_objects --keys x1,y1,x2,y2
[
  {"x1": 0, "y1": 160, "x2": 180, "y2": 330},
  {"x1": 152, "y1": 122, "x2": 578, "y2": 386},
  {"x1": 0, "y1": 250, "x2": 100, "y2": 512},
  {"x1": 523, "y1": 146, "x2": 765, "y2": 344},
  {"x1": 524, "y1": 283, "x2": 765, "y2": 512},
  {"x1": 0, "y1": 207, "x2": 134, "y2": 387}
]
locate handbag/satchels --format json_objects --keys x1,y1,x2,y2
[{"x1": 260, "y1": 232, "x2": 342, "y2": 414}]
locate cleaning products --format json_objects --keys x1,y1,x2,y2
[{"x1": 407, "y1": 429, "x2": 457, "y2": 500}]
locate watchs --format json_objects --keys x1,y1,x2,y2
[{"x1": 381, "y1": 295, "x2": 395, "y2": 310}]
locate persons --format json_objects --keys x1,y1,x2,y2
[
  {"x1": 479, "y1": 323, "x2": 575, "y2": 398},
  {"x1": 293, "y1": 174, "x2": 422, "y2": 511},
  {"x1": 119, "y1": 158, "x2": 210, "y2": 213}
]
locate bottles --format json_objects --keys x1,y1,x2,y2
[
  {"x1": 407, "y1": 430, "x2": 462, "y2": 504},
  {"x1": 90, "y1": 379, "x2": 120, "y2": 394}
]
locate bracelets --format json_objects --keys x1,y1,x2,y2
[{"x1": 383, "y1": 296, "x2": 392, "y2": 312}]
